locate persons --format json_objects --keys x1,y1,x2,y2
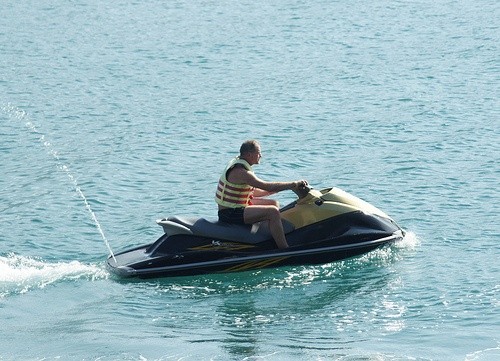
[{"x1": 215, "y1": 141, "x2": 308, "y2": 249}]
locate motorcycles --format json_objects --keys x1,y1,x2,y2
[{"x1": 104, "y1": 184, "x2": 406, "y2": 278}]
